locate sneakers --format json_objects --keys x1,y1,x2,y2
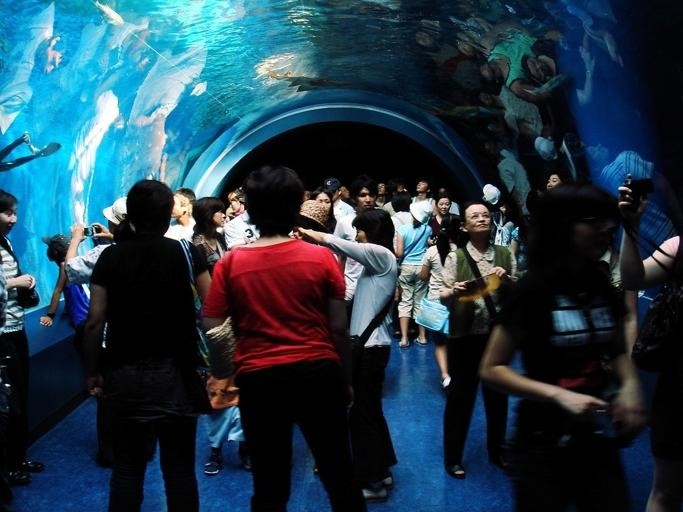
[
  {"x1": 240, "y1": 454, "x2": 253, "y2": 470},
  {"x1": 361, "y1": 469, "x2": 393, "y2": 502},
  {"x1": 440, "y1": 375, "x2": 453, "y2": 391},
  {"x1": 203, "y1": 455, "x2": 223, "y2": 475}
]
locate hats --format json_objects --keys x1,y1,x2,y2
[
  {"x1": 103, "y1": 194, "x2": 136, "y2": 233},
  {"x1": 482, "y1": 183, "x2": 501, "y2": 205},
  {"x1": 325, "y1": 178, "x2": 342, "y2": 192},
  {"x1": 534, "y1": 137, "x2": 557, "y2": 160},
  {"x1": 410, "y1": 199, "x2": 432, "y2": 227},
  {"x1": 297, "y1": 200, "x2": 329, "y2": 232}
]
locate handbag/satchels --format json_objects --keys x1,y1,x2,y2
[
  {"x1": 192, "y1": 281, "x2": 211, "y2": 370},
  {"x1": 415, "y1": 297, "x2": 448, "y2": 333},
  {"x1": 572, "y1": 390, "x2": 643, "y2": 450},
  {"x1": 16, "y1": 275, "x2": 39, "y2": 308},
  {"x1": 631, "y1": 282, "x2": 683, "y2": 374},
  {"x1": 350, "y1": 336, "x2": 364, "y2": 369},
  {"x1": 206, "y1": 315, "x2": 239, "y2": 380}
]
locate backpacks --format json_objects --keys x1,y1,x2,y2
[{"x1": 491, "y1": 211, "x2": 505, "y2": 246}]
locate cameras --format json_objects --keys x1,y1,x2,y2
[
  {"x1": 84, "y1": 226, "x2": 97, "y2": 236},
  {"x1": 624, "y1": 179, "x2": 654, "y2": 196}
]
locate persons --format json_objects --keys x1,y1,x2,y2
[
  {"x1": 0, "y1": 1, "x2": 219, "y2": 215},
  {"x1": 0, "y1": 186, "x2": 44, "y2": 486},
  {"x1": 37, "y1": 168, "x2": 683, "y2": 512},
  {"x1": 408, "y1": 0, "x2": 658, "y2": 164}
]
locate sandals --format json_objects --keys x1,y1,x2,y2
[
  {"x1": 445, "y1": 459, "x2": 466, "y2": 480},
  {"x1": 18, "y1": 459, "x2": 45, "y2": 473},
  {"x1": 5, "y1": 471, "x2": 33, "y2": 486},
  {"x1": 488, "y1": 454, "x2": 511, "y2": 470}
]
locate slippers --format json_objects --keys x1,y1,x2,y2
[{"x1": 392, "y1": 328, "x2": 428, "y2": 349}]
